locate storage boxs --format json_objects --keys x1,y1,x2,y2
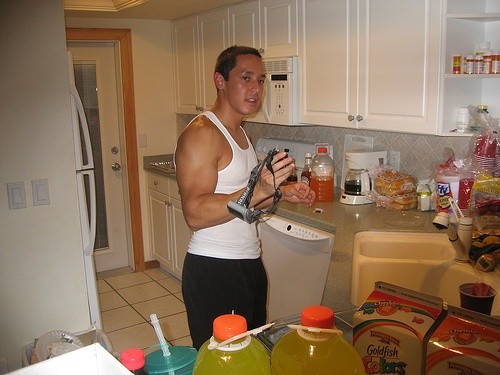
[{"x1": 352, "y1": 280, "x2": 500, "y2": 375}]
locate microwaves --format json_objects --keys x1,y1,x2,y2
[{"x1": 242, "y1": 55, "x2": 301, "y2": 126}]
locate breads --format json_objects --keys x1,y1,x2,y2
[{"x1": 373, "y1": 170, "x2": 418, "y2": 210}]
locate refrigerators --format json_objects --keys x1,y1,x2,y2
[{"x1": 0, "y1": 0, "x2": 103, "y2": 375}]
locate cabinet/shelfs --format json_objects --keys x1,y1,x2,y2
[
  {"x1": 170, "y1": 0, "x2": 500, "y2": 137},
  {"x1": 144, "y1": 170, "x2": 195, "y2": 282}
]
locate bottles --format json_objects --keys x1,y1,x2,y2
[
  {"x1": 458, "y1": 131, "x2": 500, "y2": 217},
  {"x1": 271, "y1": 306, "x2": 368, "y2": 375},
  {"x1": 191, "y1": 313, "x2": 271, "y2": 375},
  {"x1": 477, "y1": 105, "x2": 490, "y2": 115},
  {"x1": 121, "y1": 349, "x2": 147, "y2": 375},
  {"x1": 451, "y1": 41, "x2": 500, "y2": 75},
  {"x1": 310, "y1": 146, "x2": 335, "y2": 204},
  {"x1": 418, "y1": 191, "x2": 430, "y2": 211},
  {"x1": 282, "y1": 148, "x2": 297, "y2": 183},
  {"x1": 457, "y1": 107, "x2": 470, "y2": 132},
  {"x1": 300, "y1": 152, "x2": 313, "y2": 187}
]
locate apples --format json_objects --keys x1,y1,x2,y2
[{"x1": 471, "y1": 229, "x2": 500, "y2": 272}]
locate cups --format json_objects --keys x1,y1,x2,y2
[
  {"x1": 142, "y1": 345, "x2": 198, "y2": 375},
  {"x1": 458, "y1": 283, "x2": 496, "y2": 316}
]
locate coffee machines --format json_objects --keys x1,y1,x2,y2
[{"x1": 338, "y1": 149, "x2": 388, "y2": 206}]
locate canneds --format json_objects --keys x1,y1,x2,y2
[{"x1": 453, "y1": 53, "x2": 500, "y2": 74}]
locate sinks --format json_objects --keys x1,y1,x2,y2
[
  {"x1": 350, "y1": 260, "x2": 500, "y2": 316},
  {"x1": 353, "y1": 230, "x2": 454, "y2": 259}
]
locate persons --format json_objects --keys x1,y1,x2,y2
[{"x1": 173, "y1": 46, "x2": 316, "y2": 353}]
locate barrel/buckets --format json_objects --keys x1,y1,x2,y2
[{"x1": 435, "y1": 171, "x2": 465, "y2": 213}]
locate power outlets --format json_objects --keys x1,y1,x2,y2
[{"x1": 390, "y1": 151, "x2": 400, "y2": 172}]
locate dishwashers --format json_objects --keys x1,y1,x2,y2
[{"x1": 256, "y1": 212, "x2": 336, "y2": 329}]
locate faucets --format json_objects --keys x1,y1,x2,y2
[{"x1": 431, "y1": 197, "x2": 473, "y2": 264}]
